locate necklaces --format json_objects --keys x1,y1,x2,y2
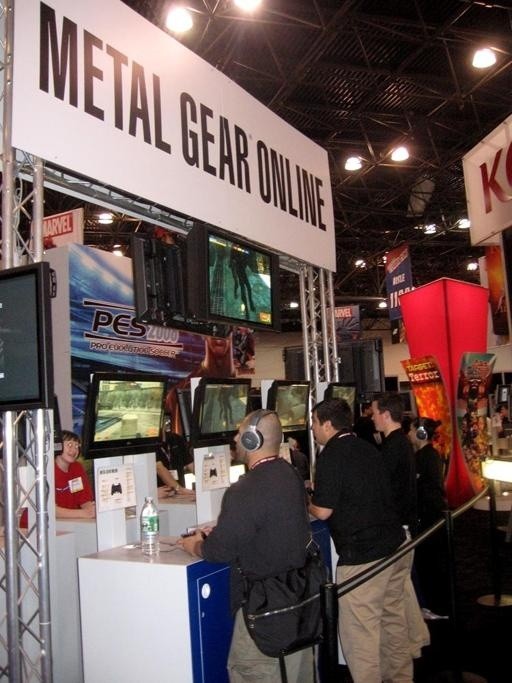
[{"x1": 159, "y1": 444, "x2": 171, "y2": 461}]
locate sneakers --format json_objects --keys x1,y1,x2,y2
[{"x1": 419, "y1": 607, "x2": 452, "y2": 623}]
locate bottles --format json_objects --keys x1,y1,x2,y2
[{"x1": 139, "y1": 496, "x2": 161, "y2": 560}]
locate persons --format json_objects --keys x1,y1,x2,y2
[
  {"x1": 407, "y1": 414, "x2": 459, "y2": 622},
  {"x1": 499, "y1": 401, "x2": 512, "y2": 423},
  {"x1": 217, "y1": 386, "x2": 234, "y2": 426},
  {"x1": 54, "y1": 430, "x2": 96, "y2": 520},
  {"x1": 228, "y1": 241, "x2": 258, "y2": 318},
  {"x1": 178, "y1": 407, "x2": 322, "y2": 683},
  {"x1": 303, "y1": 395, "x2": 416, "y2": 682},
  {"x1": 156, "y1": 411, "x2": 195, "y2": 497},
  {"x1": 370, "y1": 390, "x2": 420, "y2": 537},
  {"x1": 165, "y1": 336, "x2": 233, "y2": 438}
]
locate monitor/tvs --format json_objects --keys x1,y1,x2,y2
[
  {"x1": 260, "y1": 375, "x2": 311, "y2": 435},
  {"x1": 248, "y1": 385, "x2": 262, "y2": 418},
  {"x1": 184, "y1": 219, "x2": 284, "y2": 336},
  {"x1": 175, "y1": 383, "x2": 191, "y2": 439},
  {"x1": 0, "y1": 260, "x2": 56, "y2": 414},
  {"x1": 324, "y1": 382, "x2": 357, "y2": 424},
  {"x1": 192, "y1": 376, "x2": 250, "y2": 447},
  {"x1": 79, "y1": 372, "x2": 169, "y2": 458},
  {"x1": 131, "y1": 235, "x2": 232, "y2": 340}
]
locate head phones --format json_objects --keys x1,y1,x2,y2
[
  {"x1": 242, "y1": 407, "x2": 277, "y2": 452},
  {"x1": 415, "y1": 417, "x2": 428, "y2": 440}
]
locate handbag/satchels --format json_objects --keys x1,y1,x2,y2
[{"x1": 240, "y1": 551, "x2": 333, "y2": 660}]
